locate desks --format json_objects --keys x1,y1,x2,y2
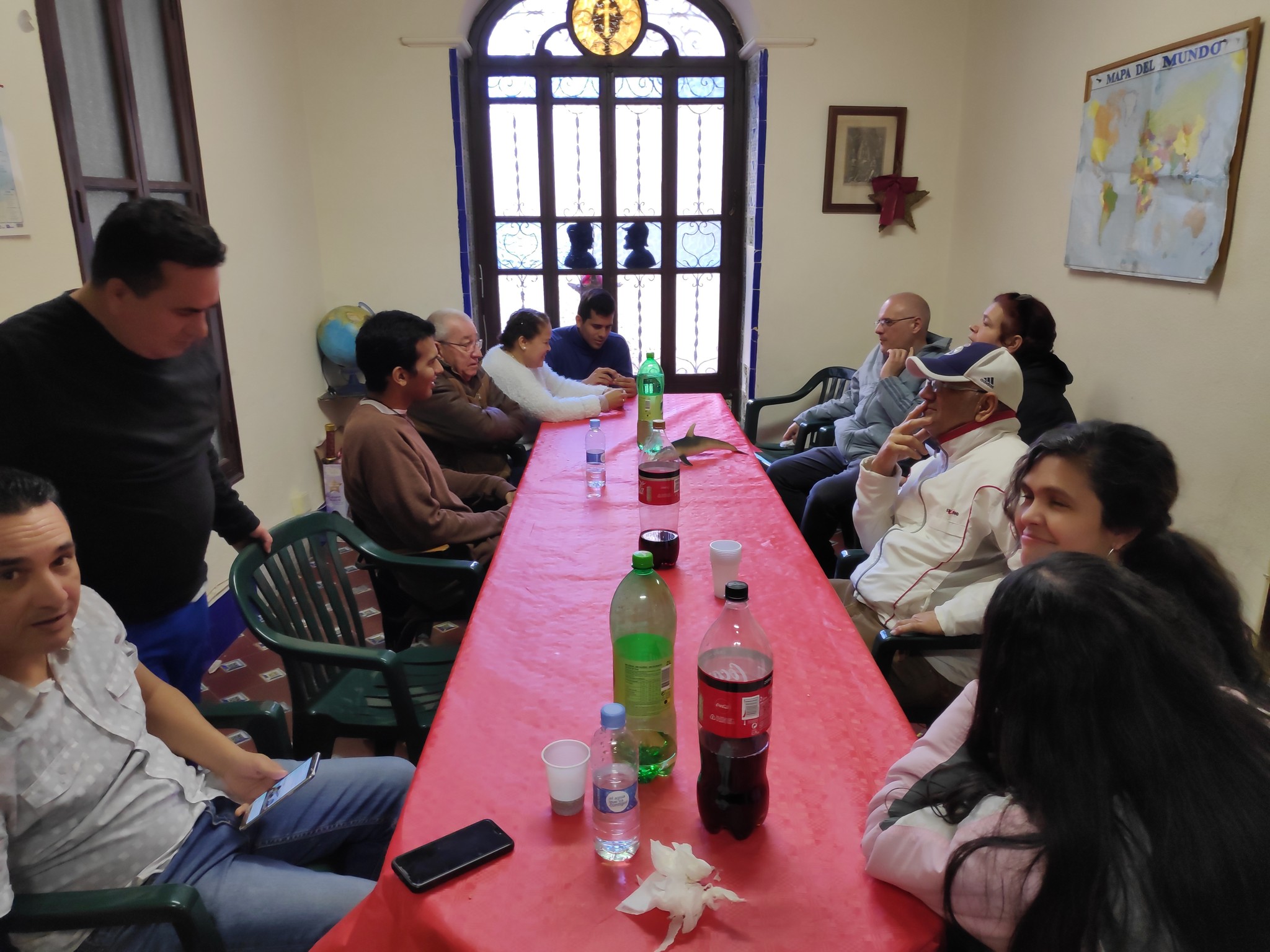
[{"x1": 338, "y1": 393, "x2": 918, "y2": 952}]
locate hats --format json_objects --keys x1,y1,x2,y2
[{"x1": 905, "y1": 342, "x2": 1024, "y2": 413}]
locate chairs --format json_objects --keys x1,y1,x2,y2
[
  {"x1": 0, "y1": 513, "x2": 481, "y2": 952},
  {"x1": 745, "y1": 366, "x2": 983, "y2": 678}
]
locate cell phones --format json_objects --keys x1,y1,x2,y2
[
  {"x1": 391, "y1": 819, "x2": 515, "y2": 894},
  {"x1": 239, "y1": 752, "x2": 322, "y2": 831}
]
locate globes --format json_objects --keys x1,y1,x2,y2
[{"x1": 317, "y1": 302, "x2": 376, "y2": 394}]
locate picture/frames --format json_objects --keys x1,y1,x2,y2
[{"x1": 823, "y1": 104, "x2": 907, "y2": 215}]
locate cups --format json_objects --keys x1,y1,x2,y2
[
  {"x1": 710, "y1": 540, "x2": 742, "y2": 599},
  {"x1": 581, "y1": 462, "x2": 606, "y2": 499},
  {"x1": 541, "y1": 739, "x2": 590, "y2": 816}
]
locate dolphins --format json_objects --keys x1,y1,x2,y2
[{"x1": 653, "y1": 423, "x2": 750, "y2": 466}]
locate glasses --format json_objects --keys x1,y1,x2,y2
[
  {"x1": 874, "y1": 317, "x2": 914, "y2": 328},
  {"x1": 438, "y1": 338, "x2": 483, "y2": 352},
  {"x1": 925, "y1": 379, "x2": 1002, "y2": 404}
]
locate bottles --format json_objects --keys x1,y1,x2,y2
[
  {"x1": 696, "y1": 580, "x2": 774, "y2": 840},
  {"x1": 585, "y1": 419, "x2": 606, "y2": 487},
  {"x1": 638, "y1": 419, "x2": 680, "y2": 570},
  {"x1": 636, "y1": 352, "x2": 664, "y2": 453},
  {"x1": 590, "y1": 703, "x2": 640, "y2": 862},
  {"x1": 609, "y1": 551, "x2": 677, "y2": 785}
]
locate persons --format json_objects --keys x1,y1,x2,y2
[
  {"x1": 404, "y1": 311, "x2": 533, "y2": 514},
  {"x1": 968, "y1": 294, "x2": 1077, "y2": 446},
  {"x1": 829, "y1": 343, "x2": 1031, "y2": 707},
  {"x1": 0, "y1": 202, "x2": 274, "y2": 771},
  {"x1": 0, "y1": 475, "x2": 416, "y2": 952},
  {"x1": 766, "y1": 293, "x2": 950, "y2": 579},
  {"x1": 544, "y1": 288, "x2": 638, "y2": 397},
  {"x1": 481, "y1": 309, "x2": 627, "y2": 422},
  {"x1": 862, "y1": 550, "x2": 1270, "y2": 952},
  {"x1": 341, "y1": 311, "x2": 519, "y2": 611},
  {"x1": 1005, "y1": 422, "x2": 1269, "y2": 697}
]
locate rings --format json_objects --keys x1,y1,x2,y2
[{"x1": 622, "y1": 399, "x2": 625, "y2": 403}]
